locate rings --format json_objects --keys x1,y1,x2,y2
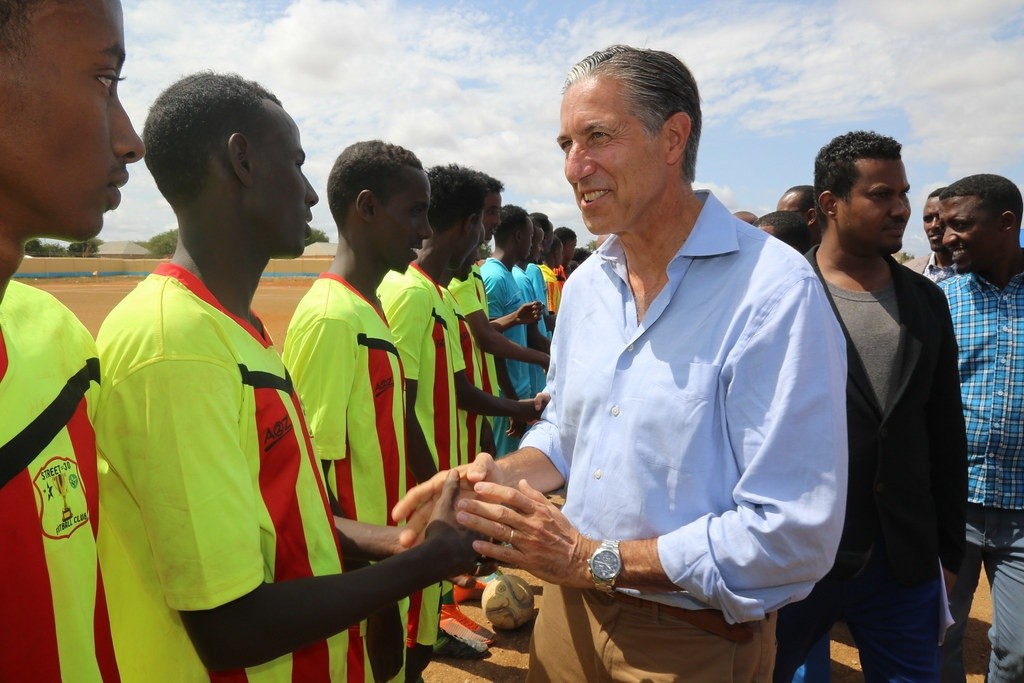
[{"x1": 508, "y1": 529, "x2": 514, "y2": 543}]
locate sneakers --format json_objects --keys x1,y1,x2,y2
[
  {"x1": 441, "y1": 603, "x2": 497, "y2": 645},
  {"x1": 435, "y1": 627, "x2": 490, "y2": 659},
  {"x1": 454, "y1": 578, "x2": 487, "y2": 604}
]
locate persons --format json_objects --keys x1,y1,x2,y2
[
  {"x1": 936, "y1": 174, "x2": 1024, "y2": 683},
  {"x1": 95, "y1": 73, "x2": 489, "y2": 683},
  {"x1": 448, "y1": 172, "x2": 592, "y2": 458},
  {"x1": 904, "y1": 188, "x2": 957, "y2": 283},
  {"x1": 281, "y1": 140, "x2": 433, "y2": 683},
  {"x1": 734, "y1": 184, "x2": 821, "y2": 255},
  {"x1": 774, "y1": 132, "x2": 969, "y2": 683},
  {"x1": 392, "y1": 45, "x2": 849, "y2": 683},
  {"x1": 0, "y1": 0, "x2": 145, "y2": 683},
  {"x1": 377, "y1": 166, "x2": 487, "y2": 683}
]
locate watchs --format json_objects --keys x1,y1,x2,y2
[{"x1": 587, "y1": 540, "x2": 622, "y2": 594}]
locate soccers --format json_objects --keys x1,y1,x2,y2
[{"x1": 481, "y1": 574, "x2": 535, "y2": 629}]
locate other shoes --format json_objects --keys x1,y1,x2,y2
[{"x1": 477, "y1": 568, "x2": 505, "y2": 584}]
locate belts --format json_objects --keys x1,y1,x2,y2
[{"x1": 594, "y1": 588, "x2": 756, "y2": 644}]
